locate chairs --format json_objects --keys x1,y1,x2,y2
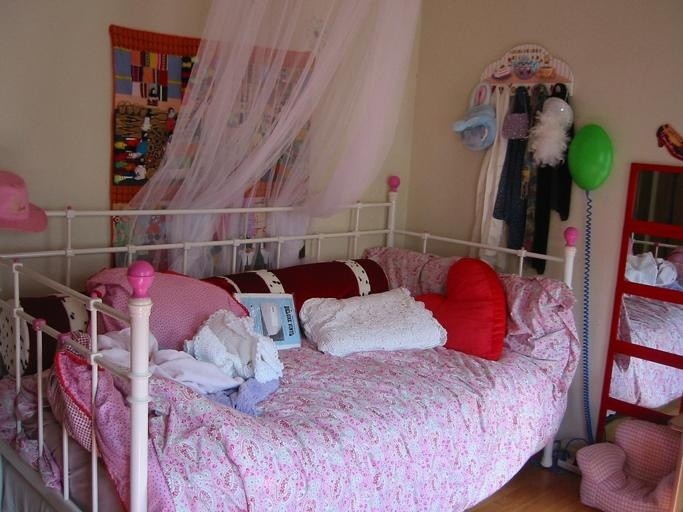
[{"x1": 576, "y1": 419, "x2": 682, "y2": 511}]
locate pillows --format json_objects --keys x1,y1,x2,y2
[
  {"x1": 412, "y1": 258, "x2": 511, "y2": 362},
  {"x1": 0, "y1": 257, "x2": 389, "y2": 380},
  {"x1": 298, "y1": 285, "x2": 449, "y2": 356}
]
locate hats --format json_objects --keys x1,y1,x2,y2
[{"x1": 0, "y1": 168, "x2": 49, "y2": 234}]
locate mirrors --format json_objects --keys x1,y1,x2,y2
[{"x1": 596, "y1": 162, "x2": 683, "y2": 442}]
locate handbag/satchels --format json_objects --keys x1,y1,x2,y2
[
  {"x1": 452, "y1": 82, "x2": 497, "y2": 151},
  {"x1": 498, "y1": 86, "x2": 531, "y2": 141}
]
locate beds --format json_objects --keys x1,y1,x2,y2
[{"x1": 1, "y1": 174, "x2": 580, "y2": 512}]
